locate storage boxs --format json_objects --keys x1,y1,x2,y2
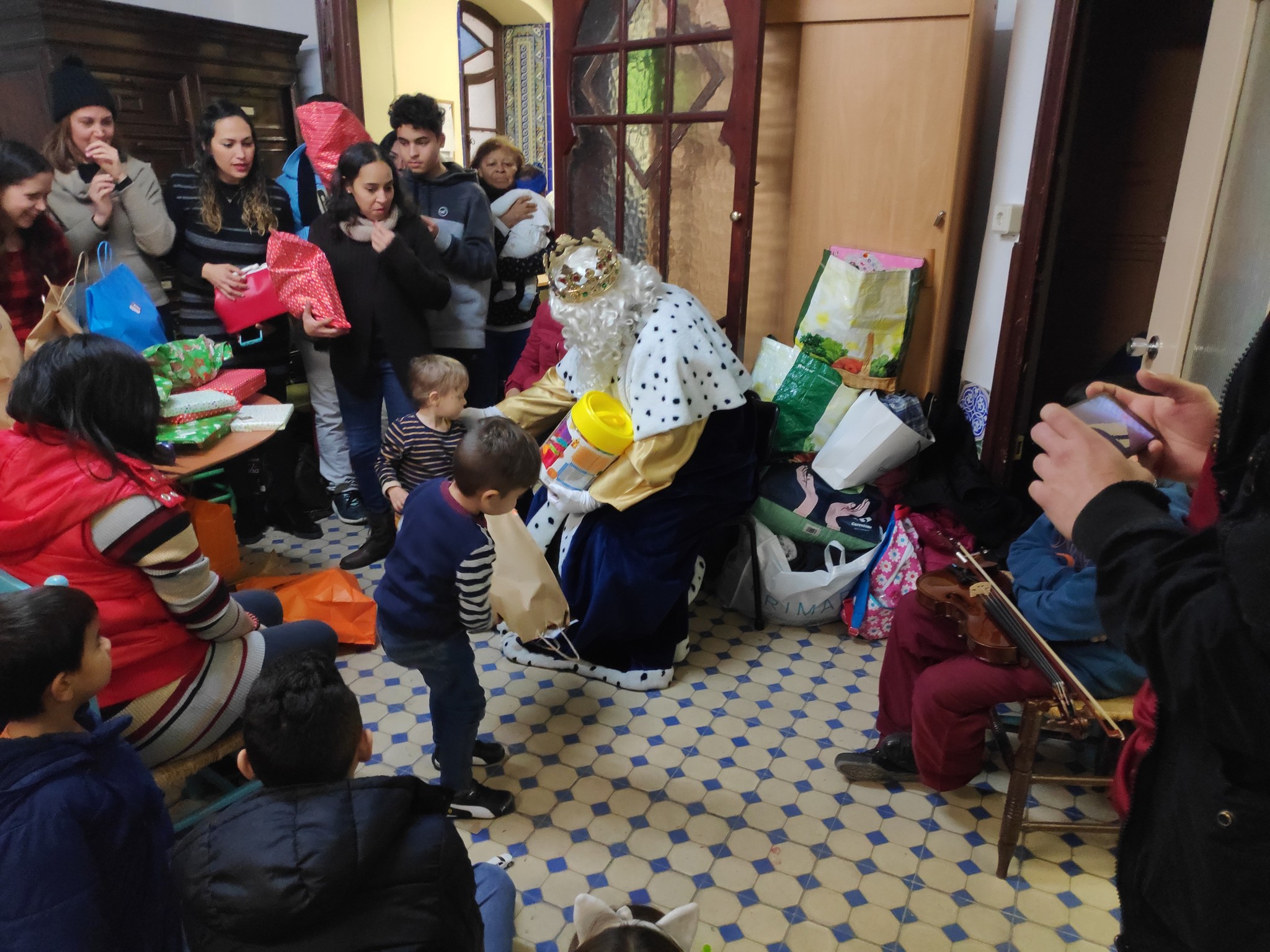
[{"x1": 192, "y1": 368, "x2": 266, "y2": 402}]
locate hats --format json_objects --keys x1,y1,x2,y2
[{"x1": 49, "y1": 55, "x2": 118, "y2": 120}]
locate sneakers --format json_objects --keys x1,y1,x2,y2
[
  {"x1": 330, "y1": 491, "x2": 368, "y2": 522},
  {"x1": 440, "y1": 780, "x2": 515, "y2": 818},
  {"x1": 432, "y1": 739, "x2": 505, "y2": 771}
]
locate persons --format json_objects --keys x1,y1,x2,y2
[
  {"x1": 564, "y1": 892, "x2": 699, "y2": 952},
  {"x1": 0, "y1": 584, "x2": 181, "y2": 952},
  {"x1": 377, "y1": 414, "x2": 543, "y2": 816},
  {"x1": 165, "y1": 650, "x2": 516, "y2": 951},
  {"x1": 0, "y1": 52, "x2": 758, "y2": 807},
  {"x1": 834, "y1": 375, "x2": 1191, "y2": 792},
  {"x1": 1026, "y1": 297, "x2": 1270, "y2": 952}
]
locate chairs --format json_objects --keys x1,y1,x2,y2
[
  {"x1": 0, "y1": 570, "x2": 245, "y2": 812},
  {"x1": 712, "y1": 389, "x2": 781, "y2": 631},
  {"x1": 989, "y1": 694, "x2": 1135, "y2": 879}
]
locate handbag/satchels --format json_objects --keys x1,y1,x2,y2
[
  {"x1": 22, "y1": 250, "x2": 89, "y2": 360},
  {"x1": 717, "y1": 239, "x2": 975, "y2": 641},
  {"x1": 485, "y1": 508, "x2": 581, "y2": 661},
  {"x1": 85, "y1": 242, "x2": 169, "y2": 355}
]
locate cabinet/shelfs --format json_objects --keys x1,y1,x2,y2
[{"x1": 1, "y1": 0, "x2": 308, "y2": 292}]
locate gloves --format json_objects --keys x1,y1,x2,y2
[
  {"x1": 457, "y1": 407, "x2": 486, "y2": 433},
  {"x1": 548, "y1": 483, "x2": 604, "y2": 514}
]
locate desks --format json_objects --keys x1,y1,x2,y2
[{"x1": 171, "y1": 391, "x2": 282, "y2": 479}]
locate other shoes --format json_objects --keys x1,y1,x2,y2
[
  {"x1": 278, "y1": 509, "x2": 323, "y2": 540},
  {"x1": 339, "y1": 537, "x2": 394, "y2": 569},
  {"x1": 834, "y1": 748, "x2": 920, "y2": 785},
  {"x1": 239, "y1": 515, "x2": 265, "y2": 546},
  {"x1": 871, "y1": 730, "x2": 918, "y2": 772}
]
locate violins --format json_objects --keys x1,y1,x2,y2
[{"x1": 915, "y1": 561, "x2": 1099, "y2": 741}]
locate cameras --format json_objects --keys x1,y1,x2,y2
[{"x1": 1069, "y1": 390, "x2": 1159, "y2": 457}]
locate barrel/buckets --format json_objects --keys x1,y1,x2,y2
[{"x1": 536, "y1": 390, "x2": 634, "y2": 495}]
